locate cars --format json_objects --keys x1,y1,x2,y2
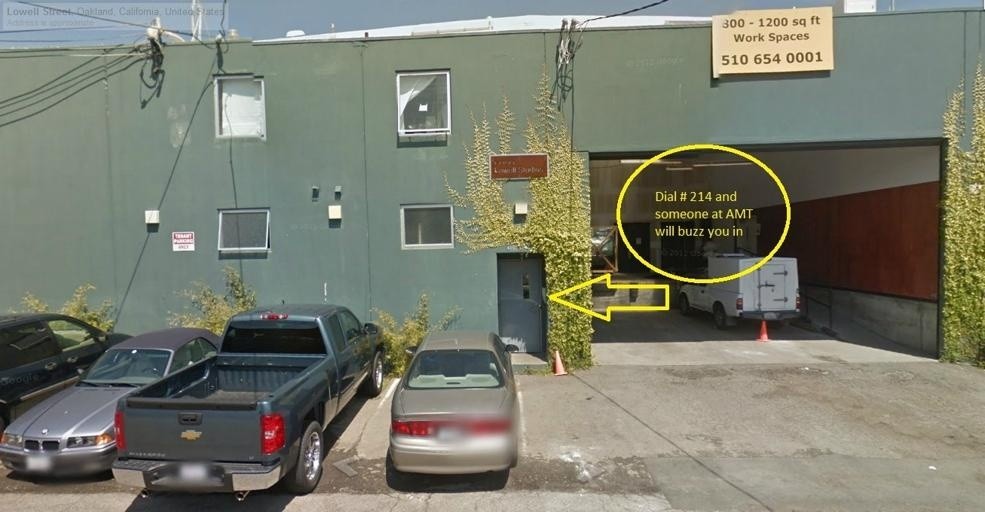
[{"x1": 2, "y1": 328, "x2": 221, "y2": 479}]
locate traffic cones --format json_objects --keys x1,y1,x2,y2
[
  {"x1": 552, "y1": 350, "x2": 568, "y2": 377},
  {"x1": 756, "y1": 320, "x2": 769, "y2": 343}
]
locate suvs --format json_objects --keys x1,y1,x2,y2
[
  {"x1": 390, "y1": 331, "x2": 522, "y2": 475},
  {"x1": 0, "y1": 312, "x2": 134, "y2": 429}
]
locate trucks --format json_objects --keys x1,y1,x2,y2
[
  {"x1": 110, "y1": 302, "x2": 386, "y2": 500},
  {"x1": 678, "y1": 252, "x2": 801, "y2": 328}
]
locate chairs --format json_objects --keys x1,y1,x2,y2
[
  {"x1": 173, "y1": 349, "x2": 191, "y2": 367},
  {"x1": 128, "y1": 356, "x2": 152, "y2": 375}
]
